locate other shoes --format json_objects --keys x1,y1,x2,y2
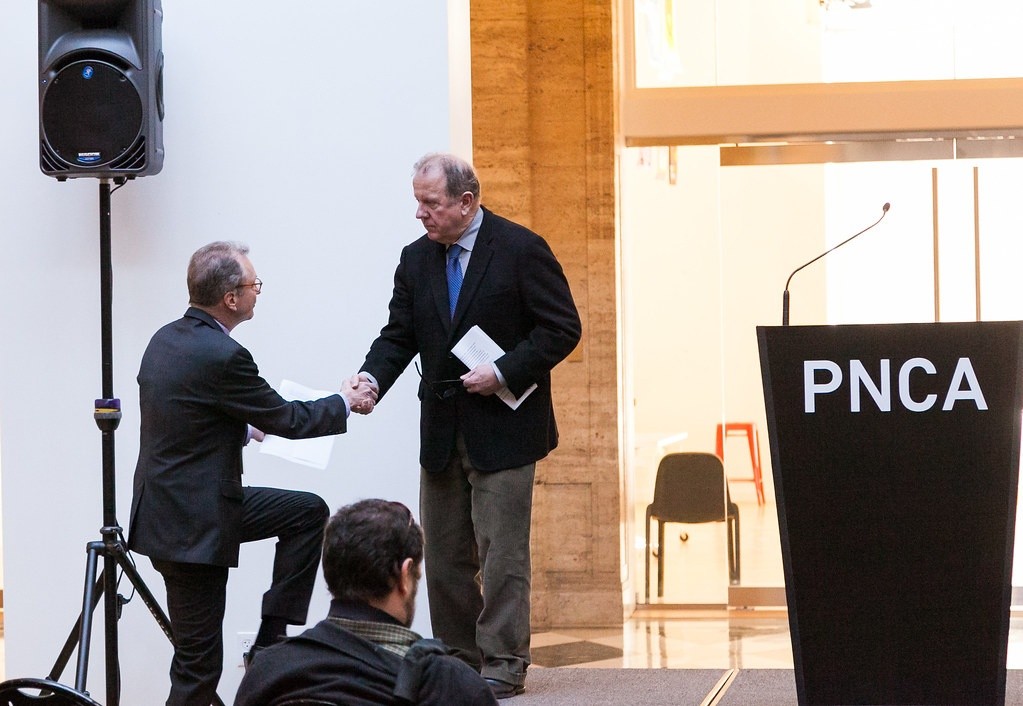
[{"x1": 248, "y1": 645, "x2": 264, "y2": 666}]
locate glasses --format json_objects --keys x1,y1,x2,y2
[
  {"x1": 231, "y1": 278, "x2": 262, "y2": 294},
  {"x1": 415, "y1": 361, "x2": 466, "y2": 400}
]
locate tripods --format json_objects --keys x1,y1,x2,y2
[{"x1": 38, "y1": 178, "x2": 224, "y2": 706}]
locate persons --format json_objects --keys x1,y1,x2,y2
[
  {"x1": 350, "y1": 150, "x2": 583, "y2": 698},
  {"x1": 123, "y1": 239, "x2": 379, "y2": 706},
  {"x1": 234, "y1": 498, "x2": 502, "y2": 706}
]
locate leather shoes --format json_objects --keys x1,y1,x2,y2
[{"x1": 486, "y1": 678, "x2": 525, "y2": 698}]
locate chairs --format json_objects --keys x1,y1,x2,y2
[{"x1": 644, "y1": 452, "x2": 742, "y2": 598}]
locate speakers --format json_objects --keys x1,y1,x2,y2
[{"x1": 38, "y1": 0, "x2": 165, "y2": 182}]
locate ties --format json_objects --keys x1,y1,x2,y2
[{"x1": 447, "y1": 244, "x2": 463, "y2": 320}]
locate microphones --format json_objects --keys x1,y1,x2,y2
[{"x1": 781, "y1": 202, "x2": 890, "y2": 325}]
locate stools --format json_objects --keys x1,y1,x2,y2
[{"x1": 716, "y1": 422, "x2": 765, "y2": 505}]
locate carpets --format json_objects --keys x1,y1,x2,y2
[{"x1": 495, "y1": 666, "x2": 798, "y2": 705}]
